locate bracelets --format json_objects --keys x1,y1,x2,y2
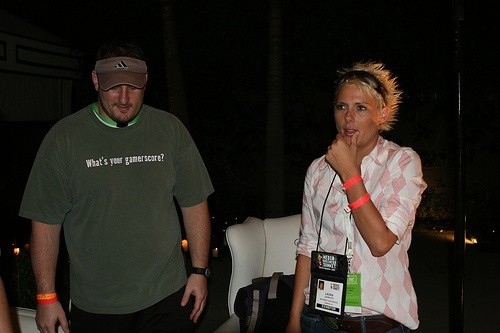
[
  {"x1": 342, "y1": 173, "x2": 363, "y2": 191},
  {"x1": 348, "y1": 193, "x2": 371, "y2": 212},
  {"x1": 35, "y1": 292, "x2": 59, "y2": 304}
]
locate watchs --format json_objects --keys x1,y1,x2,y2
[{"x1": 190, "y1": 267, "x2": 211, "y2": 278}]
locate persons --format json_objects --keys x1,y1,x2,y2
[
  {"x1": 284, "y1": 59, "x2": 428, "y2": 332},
  {"x1": 16, "y1": 42, "x2": 217, "y2": 333},
  {"x1": 0, "y1": 277, "x2": 17, "y2": 333}
]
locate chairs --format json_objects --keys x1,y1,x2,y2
[{"x1": 210, "y1": 213, "x2": 302, "y2": 333}]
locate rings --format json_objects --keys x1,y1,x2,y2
[{"x1": 327, "y1": 146, "x2": 331, "y2": 150}]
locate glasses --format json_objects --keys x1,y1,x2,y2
[{"x1": 335, "y1": 70, "x2": 388, "y2": 102}]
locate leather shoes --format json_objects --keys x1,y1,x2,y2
[{"x1": 340, "y1": 316, "x2": 401, "y2": 333}]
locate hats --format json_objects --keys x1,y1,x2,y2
[{"x1": 95, "y1": 57, "x2": 148, "y2": 92}]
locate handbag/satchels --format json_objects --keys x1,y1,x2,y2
[{"x1": 233, "y1": 271, "x2": 296, "y2": 333}]
